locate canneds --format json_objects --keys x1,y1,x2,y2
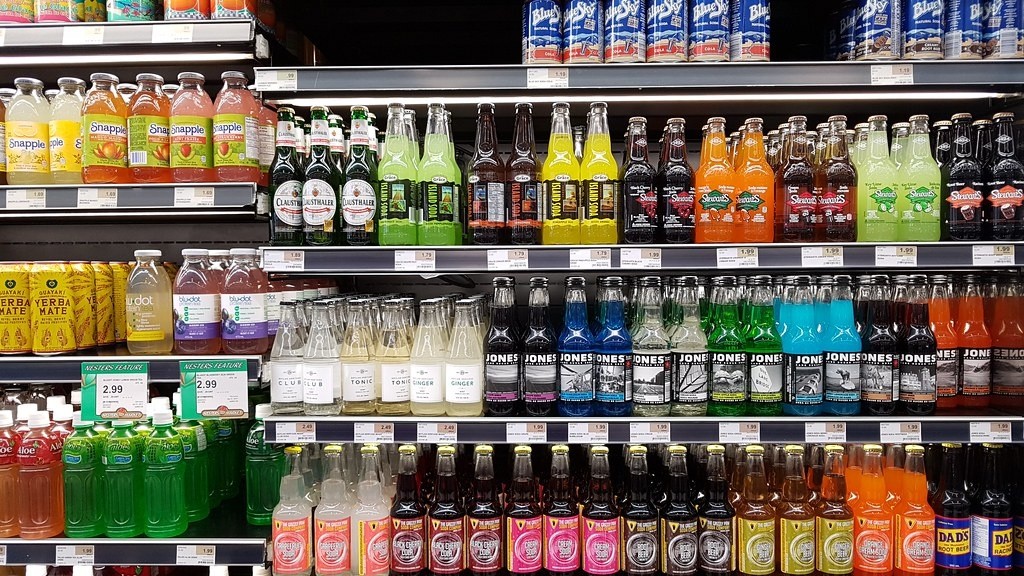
[
  {"x1": 828, "y1": 0, "x2": 1024, "y2": 61},
  {"x1": 0, "y1": 0, "x2": 326, "y2": 66},
  {"x1": 0, "y1": 260, "x2": 178, "y2": 356},
  {"x1": 522, "y1": 0, "x2": 771, "y2": 65}
]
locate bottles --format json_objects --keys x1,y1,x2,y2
[
  {"x1": 126, "y1": 248, "x2": 339, "y2": 355},
  {"x1": 268, "y1": 101, "x2": 1024, "y2": 245},
  {"x1": 272, "y1": 273, "x2": 1024, "y2": 416},
  {"x1": 0, "y1": 70, "x2": 276, "y2": 184},
  {"x1": 268, "y1": 443, "x2": 1024, "y2": 576},
  {"x1": 0, "y1": 384, "x2": 292, "y2": 540}
]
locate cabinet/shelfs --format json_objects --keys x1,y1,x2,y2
[
  {"x1": 0, "y1": 19, "x2": 267, "y2": 565},
  {"x1": 252, "y1": 58, "x2": 1024, "y2": 576}
]
